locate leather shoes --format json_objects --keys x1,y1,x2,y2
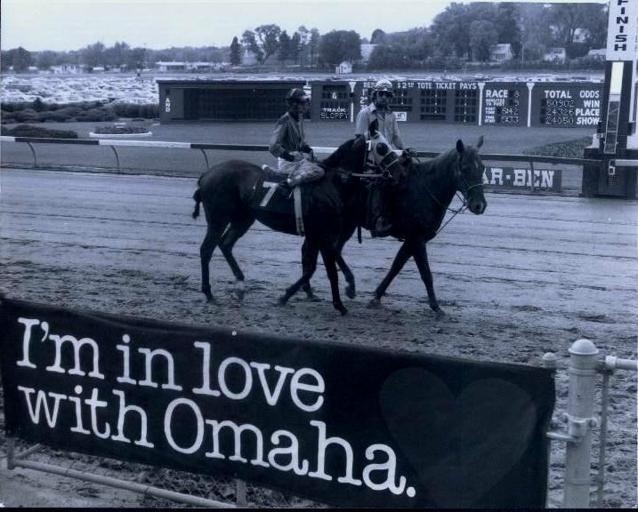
[
  {"x1": 371, "y1": 216, "x2": 394, "y2": 238},
  {"x1": 278, "y1": 179, "x2": 291, "y2": 201}
]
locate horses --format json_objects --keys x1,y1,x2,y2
[
  {"x1": 301, "y1": 136, "x2": 487, "y2": 316},
  {"x1": 192, "y1": 118, "x2": 407, "y2": 315}
]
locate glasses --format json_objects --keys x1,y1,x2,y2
[{"x1": 378, "y1": 91, "x2": 392, "y2": 98}]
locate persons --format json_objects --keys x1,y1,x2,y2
[
  {"x1": 268, "y1": 88, "x2": 326, "y2": 200},
  {"x1": 353, "y1": 78, "x2": 417, "y2": 237}
]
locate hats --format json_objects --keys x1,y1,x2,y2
[
  {"x1": 286, "y1": 88, "x2": 310, "y2": 102},
  {"x1": 375, "y1": 79, "x2": 393, "y2": 92}
]
